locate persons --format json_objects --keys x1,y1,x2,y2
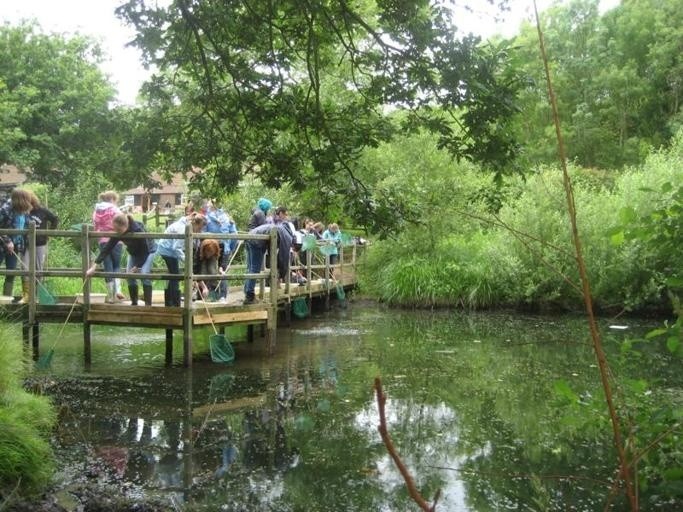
[
  {"x1": 0, "y1": 185, "x2": 34, "y2": 296},
  {"x1": 18, "y1": 190, "x2": 59, "y2": 305},
  {"x1": 83, "y1": 190, "x2": 343, "y2": 306}
]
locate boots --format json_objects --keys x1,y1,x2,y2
[
  {"x1": 163, "y1": 288, "x2": 180, "y2": 306},
  {"x1": 18, "y1": 280, "x2": 29, "y2": 304},
  {"x1": 243, "y1": 292, "x2": 259, "y2": 304},
  {"x1": 106, "y1": 278, "x2": 152, "y2": 306}
]
